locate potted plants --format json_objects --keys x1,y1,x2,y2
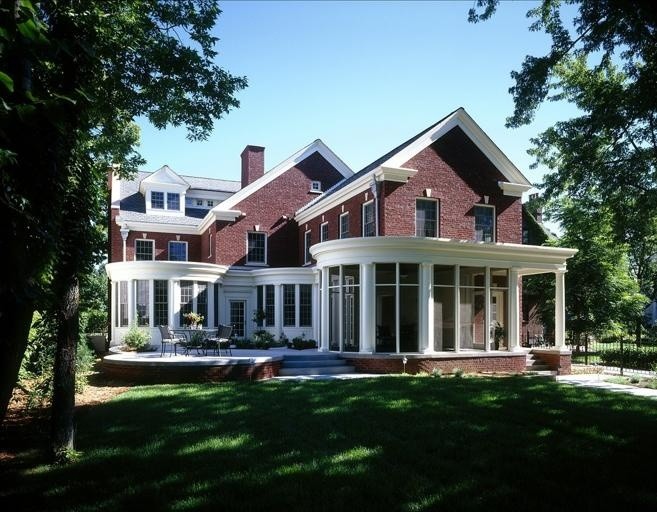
[
  {"x1": 250, "y1": 310, "x2": 275, "y2": 350},
  {"x1": 487, "y1": 322, "x2": 504, "y2": 350}
]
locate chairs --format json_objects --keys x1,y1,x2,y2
[{"x1": 158, "y1": 325, "x2": 233, "y2": 358}]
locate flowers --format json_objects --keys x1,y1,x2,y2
[{"x1": 183, "y1": 312, "x2": 205, "y2": 323}]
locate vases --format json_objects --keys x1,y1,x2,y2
[{"x1": 190, "y1": 322, "x2": 198, "y2": 328}]
